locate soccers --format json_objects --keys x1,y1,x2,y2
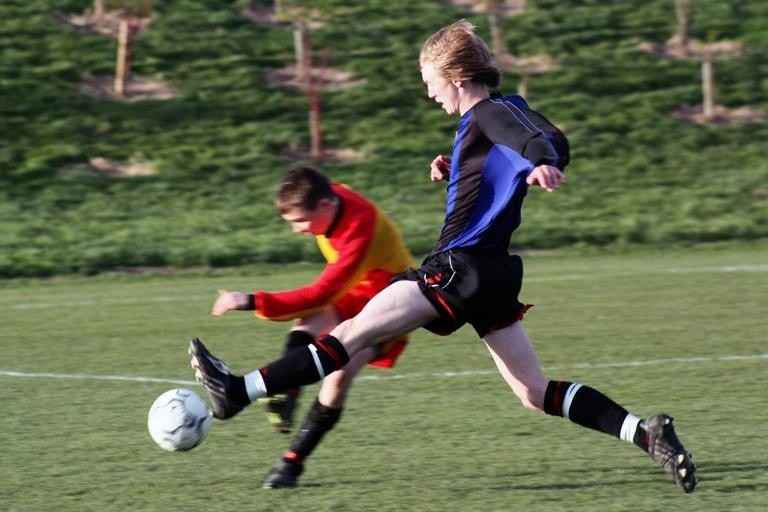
[{"x1": 147, "y1": 388, "x2": 213, "y2": 453}]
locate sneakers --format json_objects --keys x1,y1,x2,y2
[
  {"x1": 263, "y1": 459, "x2": 306, "y2": 490},
  {"x1": 187, "y1": 335, "x2": 244, "y2": 420},
  {"x1": 639, "y1": 412, "x2": 697, "y2": 493},
  {"x1": 257, "y1": 393, "x2": 295, "y2": 434}
]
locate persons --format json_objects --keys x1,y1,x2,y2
[
  {"x1": 211, "y1": 167, "x2": 413, "y2": 489},
  {"x1": 189, "y1": 20, "x2": 697, "y2": 495}
]
